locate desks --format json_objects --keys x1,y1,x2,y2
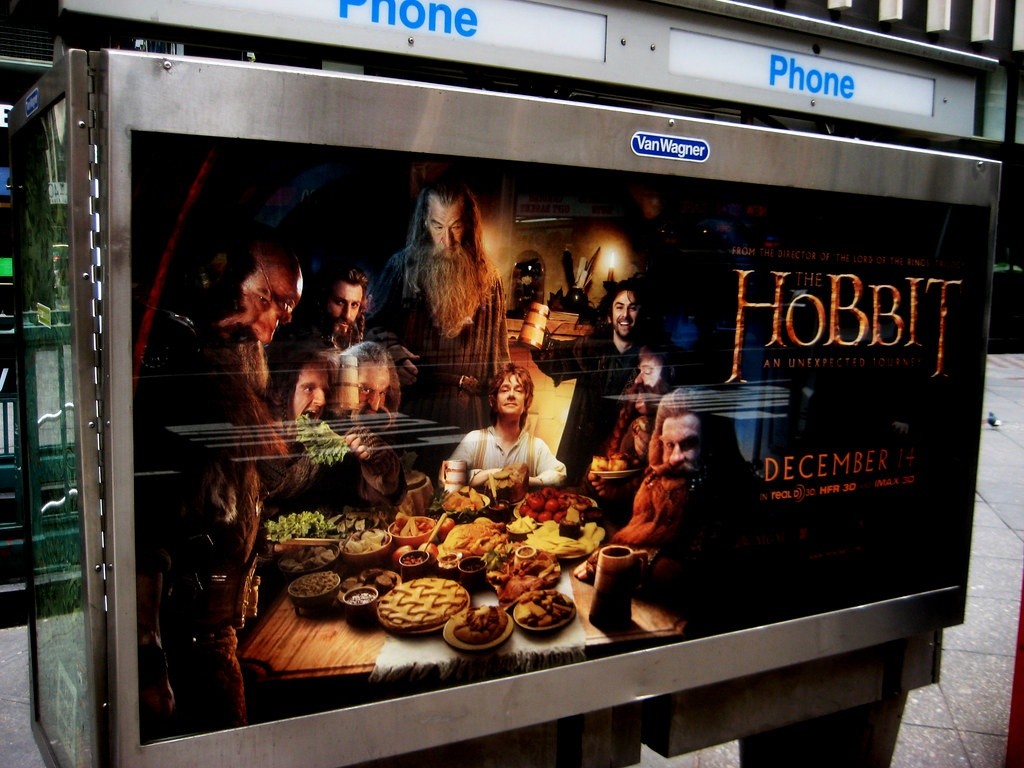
[{"x1": 237, "y1": 566, "x2": 690, "y2": 691}]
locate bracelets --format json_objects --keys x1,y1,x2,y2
[{"x1": 647, "y1": 548, "x2": 661, "y2": 567}]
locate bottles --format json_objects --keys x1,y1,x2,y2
[
  {"x1": 565, "y1": 287, "x2": 587, "y2": 325},
  {"x1": 484, "y1": 472, "x2": 515, "y2": 503},
  {"x1": 398, "y1": 471, "x2": 434, "y2": 517},
  {"x1": 437, "y1": 552, "x2": 462, "y2": 583}
]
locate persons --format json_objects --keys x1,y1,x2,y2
[{"x1": 143, "y1": 173, "x2": 912, "y2": 736}]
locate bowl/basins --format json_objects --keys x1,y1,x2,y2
[
  {"x1": 287, "y1": 572, "x2": 341, "y2": 615},
  {"x1": 278, "y1": 544, "x2": 340, "y2": 581},
  {"x1": 506, "y1": 520, "x2": 537, "y2": 542},
  {"x1": 326, "y1": 512, "x2": 379, "y2": 540},
  {"x1": 339, "y1": 528, "x2": 392, "y2": 569},
  {"x1": 388, "y1": 516, "x2": 437, "y2": 550},
  {"x1": 514, "y1": 547, "x2": 537, "y2": 569}
]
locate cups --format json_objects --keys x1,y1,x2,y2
[
  {"x1": 489, "y1": 499, "x2": 511, "y2": 523},
  {"x1": 442, "y1": 460, "x2": 465, "y2": 494},
  {"x1": 589, "y1": 545, "x2": 648, "y2": 628},
  {"x1": 459, "y1": 556, "x2": 487, "y2": 591},
  {"x1": 399, "y1": 551, "x2": 432, "y2": 584},
  {"x1": 332, "y1": 355, "x2": 359, "y2": 406},
  {"x1": 343, "y1": 588, "x2": 378, "y2": 626},
  {"x1": 518, "y1": 303, "x2": 549, "y2": 350}
]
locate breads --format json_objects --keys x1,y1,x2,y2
[{"x1": 590, "y1": 452, "x2": 630, "y2": 471}]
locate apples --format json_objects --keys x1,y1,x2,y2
[
  {"x1": 520, "y1": 487, "x2": 568, "y2": 524},
  {"x1": 392, "y1": 517, "x2": 454, "y2": 566}
]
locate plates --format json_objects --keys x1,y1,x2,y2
[
  {"x1": 513, "y1": 496, "x2": 598, "y2": 525},
  {"x1": 590, "y1": 468, "x2": 641, "y2": 478},
  {"x1": 555, "y1": 526, "x2": 597, "y2": 558},
  {"x1": 338, "y1": 570, "x2": 470, "y2": 633},
  {"x1": 514, "y1": 591, "x2": 576, "y2": 630},
  {"x1": 444, "y1": 608, "x2": 513, "y2": 650}
]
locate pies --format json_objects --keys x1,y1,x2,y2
[{"x1": 379, "y1": 577, "x2": 467, "y2": 631}]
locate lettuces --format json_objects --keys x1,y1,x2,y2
[
  {"x1": 263, "y1": 510, "x2": 339, "y2": 542},
  {"x1": 296, "y1": 412, "x2": 352, "y2": 467}
]
